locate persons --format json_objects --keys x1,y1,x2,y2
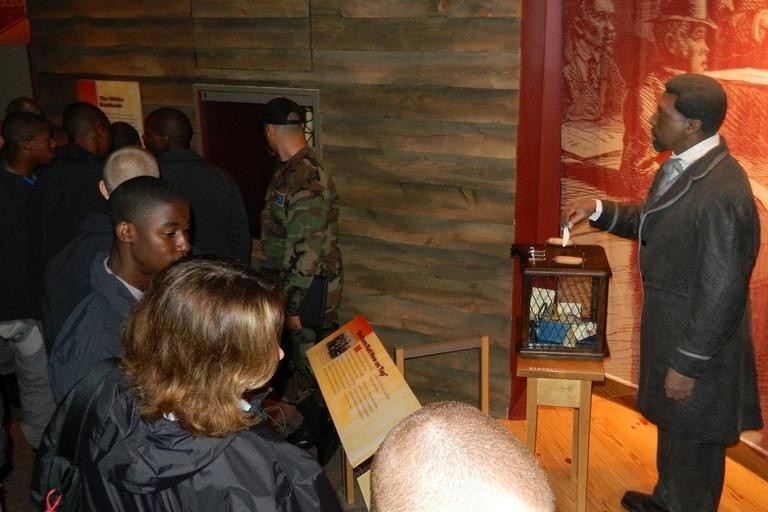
[
  {"x1": 0, "y1": 111, "x2": 57, "y2": 481},
  {"x1": 46, "y1": 175, "x2": 191, "y2": 409},
  {"x1": 710, "y1": 0, "x2": 768, "y2": 71},
  {"x1": 561, "y1": 0, "x2": 629, "y2": 241},
  {"x1": 30, "y1": 254, "x2": 344, "y2": 512},
  {"x1": 369, "y1": 399, "x2": 555, "y2": 512},
  {"x1": 6, "y1": 96, "x2": 48, "y2": 120},
  {"x1": 616, "y1": 0, "x2": 718, "y2": 386},
  {"x1": 40, "y1": 145, "x2": 161, "y2": 358},
  {"x1": 258, "y1": 96, "x2": 343, "y2": 463},
  {"x1": 142, "y1": 107, "x2": 252, "y2": 272},
  {"x1": 112, "y1": 121, "x2": 143, "y2": 151},
  {"x1": 563, "y1": 73, "x2": 763, "y2": 512},
  {"x1": 30, "y1": 100, "x2": 110, "y2": 306}
]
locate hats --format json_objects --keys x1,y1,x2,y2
[{"x1": 263, "y1": 99, "x2": 304, "y2": 124}]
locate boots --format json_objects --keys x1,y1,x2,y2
[{"x1": 287, "y1": 394, "x2": 319, "y2": 444}]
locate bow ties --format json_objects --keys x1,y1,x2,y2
[{"x1": 662, "y1": 158, "x2": 682, "y2": 173}]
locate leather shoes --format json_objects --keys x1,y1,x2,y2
[{"x1": 621, "y1": 491, "x2": 665, "y2": 512}]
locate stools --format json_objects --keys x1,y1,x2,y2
[{"x1": 515, "y1": 355, "x2": 607, "y2": 511}]
